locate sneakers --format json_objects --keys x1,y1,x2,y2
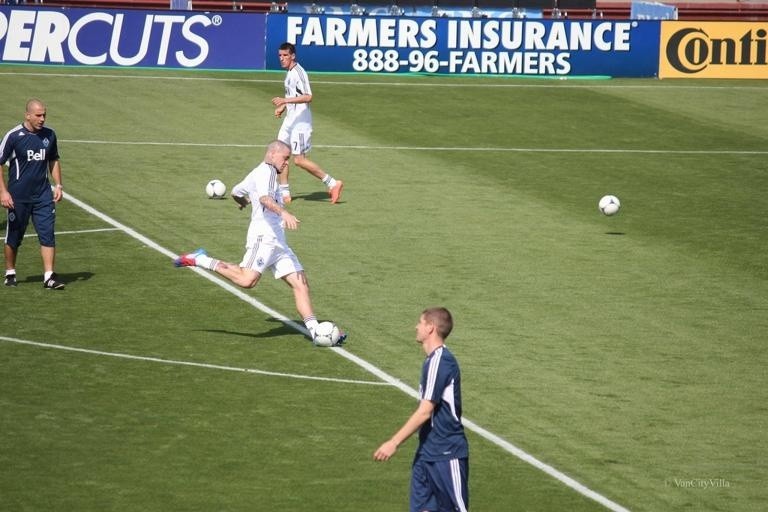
[
  {"x1": 281, "y1": 195, "x2": 291, "y2": 205},
  {"x1": 4, "y1": 274, "x2": 17, "y2": 286},
  {"x1": 329, "y1": 181, "x2": 343, "y2": 204},
  {"x1": 174, "y1": 248, "x2": 206, "y2": 267},
  {"x1": 43, "y1": 278, "x2": 63, "y2": 290}
]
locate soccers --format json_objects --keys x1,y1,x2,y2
[
  {"x1": 598, "y1": 195, "x2": 620, "y2": 216},
  {"x1": 206, "y1": 179, "x2": 226, "y2": 198}
]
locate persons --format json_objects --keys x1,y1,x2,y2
[
  {"x1": 373, "y1": 307, "x2": 469, "y2": 511},
  {"x1": 0, "y1": 99, "x2": 65, "y2": 291},
  {"x1": 272, "y1": 42, "x2": 343, "y2": 203},
  {"x1": 172, "y1": 141, "x2": 346, "y2": 343}
]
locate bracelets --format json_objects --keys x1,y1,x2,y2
[{"x1": 55, "y1": 184, "x2": 62, "y2": 189}]
